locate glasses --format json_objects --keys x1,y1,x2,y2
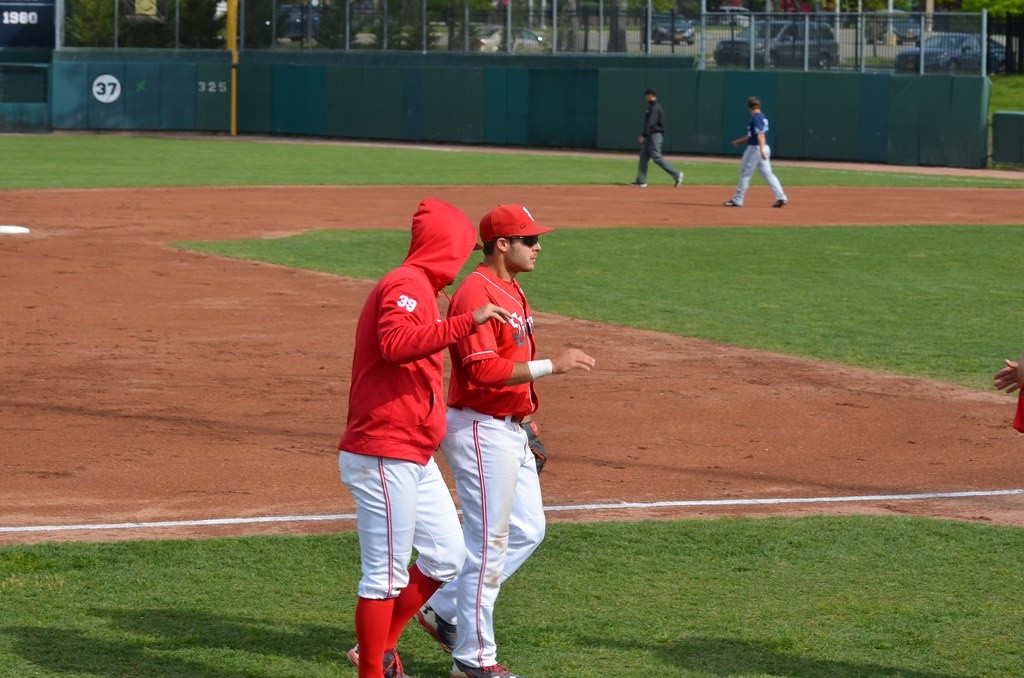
[{"x1": 495, "y1": 234, "x2": 539, "y2": 249}]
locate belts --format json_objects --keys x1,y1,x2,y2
[{"x1": 451, "y1": 404, "x2": 524, "y2": 423}]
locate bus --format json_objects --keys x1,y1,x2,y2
[{"x1": 275, "y1": 0, "x2": 327, "y2": 41}]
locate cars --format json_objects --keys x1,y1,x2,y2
[
  {"x1": 865, "y1": 11, "x2": 922, "y2": 47},
  {"x1": 705, "y1": 6, "x2": 757, "y2": 27},
  {"x1": 643, "y1": 12, "x2": 695, "y2": 45},
  {"x1": 895, "y1": 32, "x2": 1015, "y2": 77},
  {"x1": 456, "y1": 27, "x2": 553, "y2": 53}
]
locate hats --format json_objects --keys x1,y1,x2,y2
[{"x1": 479, "y1": 203, "x2": 554, "y2": 243}]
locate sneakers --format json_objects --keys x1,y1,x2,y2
[
  {"x1": 449, "y1": 658, "x2": 524, "y2": 678},
  {"x1": 347, "y1": 638, "x2": 411, "y2": 678},
  {"x1": 412, "y1": 601, "x2": 458, "y2": 653}
]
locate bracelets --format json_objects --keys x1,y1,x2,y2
[{"x1": 526, "y1": 358, "x2": 554, "y2": 379}]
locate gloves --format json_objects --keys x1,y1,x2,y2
[{"x1": 519, "y1": 420, "x2": 546, "y2": 476}]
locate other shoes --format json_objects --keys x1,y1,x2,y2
[
  {"x1": 630, "y1": 182, "x2": 648, "y2": 187},
  {"x1": 773, "y1": 200, "x2": 787, "y2": 207},
  {"x1": 674, "y1": 172, "x2": 683, "y2": 187},
  {"x1": 723, "y1": 200, "x2": 741, "y2": 207}
]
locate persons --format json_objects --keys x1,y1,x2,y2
[
  {"x1": 992, "y1": 353, "x2": 1024, "y2": 436},
  {"x1": 631, "y1": 89, "x2": 684, "y2": 188},
  {"x1": 339, "y1": 198, "x2": 514, "y2": 678},
  {"x1": 721, "y1": 95, "x2": 788, "y2": 208},
  {"x1": 412, "y1": 205, "x2": 596, "y2": 678}
]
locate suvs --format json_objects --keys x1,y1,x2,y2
[{"x1": 713, "y1": 20, "x2": 840, "y2": 70}]
